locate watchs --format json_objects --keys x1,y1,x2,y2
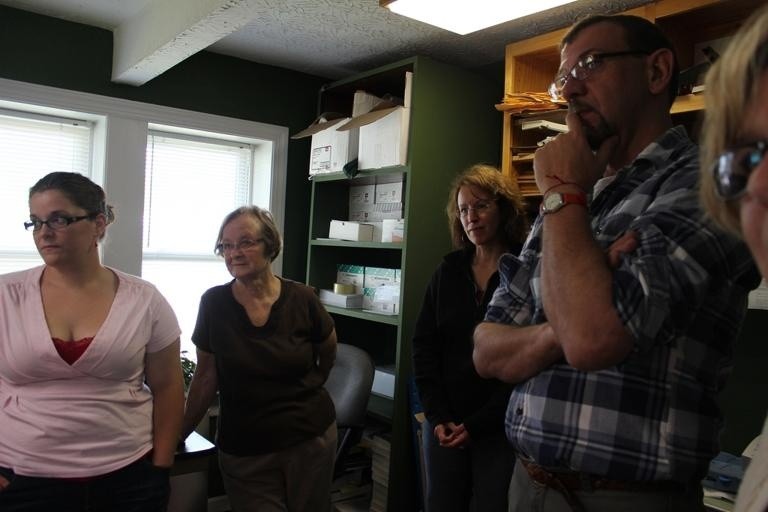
[{"x1": 539, "y1": 193, "x2": 588, "y2": 218}]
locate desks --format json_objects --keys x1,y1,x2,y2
[{"x1": 164, "y1": 425, "x2": 218, "y2": 478}]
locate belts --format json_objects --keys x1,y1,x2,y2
[{"x1": 513, "y1": 452, "x2": 702, "y2": 512}]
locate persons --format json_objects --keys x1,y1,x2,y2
[
  {"x1": 696, "y1": 2, "x2": 768, "y2": 512},
  {"x1": 181, "y1": 205, "x2": 339, "y2": 511},
  {"x1": 413, "y1": 161, "x2": 528, "y2": 512},
  {"x1": 2, "y1": 171, "x2": 185, "y2": 512},
  {"x1": 471, "y1": 14, "x2": 762, "y2": 511}
]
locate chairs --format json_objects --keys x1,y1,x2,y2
[{"x1": 313, "y1": 336, "x2": 376, "y2": 487}]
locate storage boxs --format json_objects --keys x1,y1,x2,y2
[
  {"x1": 365, "y1": 365, "x2": 398, "y2": 414},
  {"x1": 337, "y1": 65, "x2": 413, "y2": 168},
  {"x1": 314, "y1": 179, "x2": 405, "y2": 315},
  {"x1": 290, "y1": 114, "x2": 359, "y2": 174}
]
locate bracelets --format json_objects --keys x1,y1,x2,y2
[{"x1": 543, "y1": 174, "x2": 588, "y2": 194}]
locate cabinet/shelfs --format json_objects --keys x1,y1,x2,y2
[
  {"x1": 641, "y1": 1, "x2": 731, "y2": 116},
  {"x1": 303, "y1": 52, "x2": 508, "y2": 512},
  {"x1": 498, "y1": 2, "x2": 641, "y2": 202}
]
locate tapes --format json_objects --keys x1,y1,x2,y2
[{"x1": 333, "y1": 283, "x2": 355, "y2": 296}]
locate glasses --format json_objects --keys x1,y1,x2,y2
[
  {"x1": 455, "y1": 201, "x2": 499, "y2": 217},
  {"x1": 547, "y1": 49, "x2": 651, "y2": 101},
  {"x1": 215, "y1": 238, "x2": 265, "y2": 251},
  {"x1": 712, "y1": 141, "x2": 767, "y2": 201},
  {"x1": 24, "y1": 210, "x2": 101, "y2": 231}
]
